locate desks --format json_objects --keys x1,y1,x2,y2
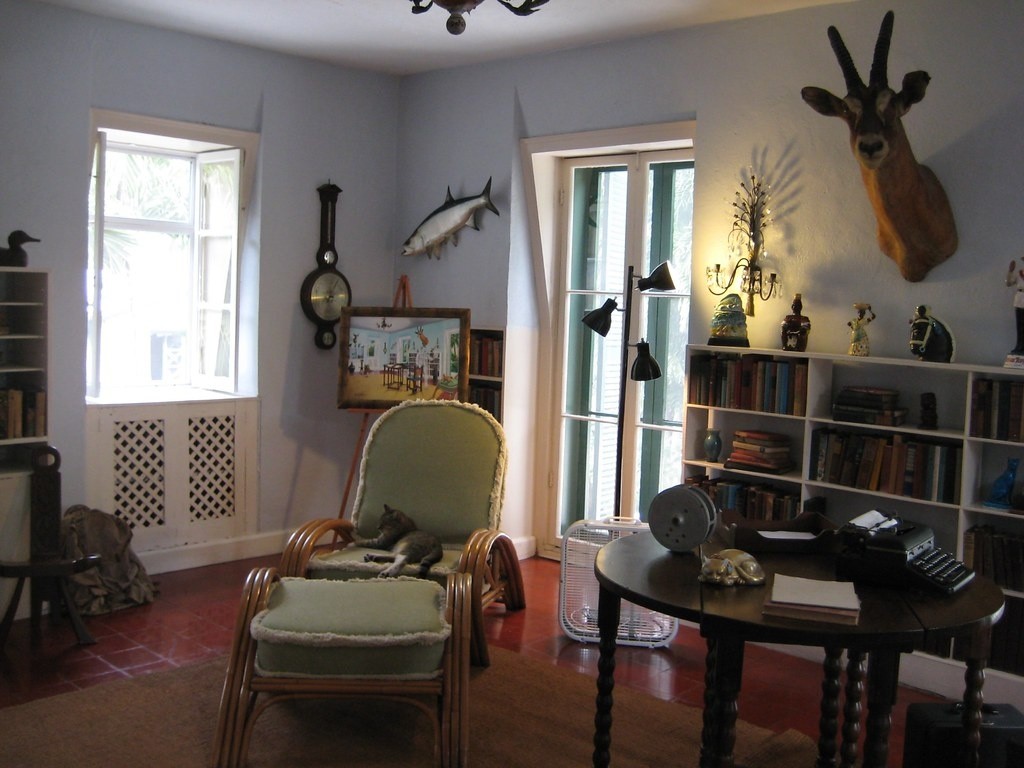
[{"x1": 593, "y1": 520, "x2": 1004, "y2": 768}]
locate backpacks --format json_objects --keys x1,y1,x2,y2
[{"x1": 59, "y1": 505, "x2": 158, "y2": 616}]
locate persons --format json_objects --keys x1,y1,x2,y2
[
  {"x1": 909, "y1": 305, "x2": 935, "y2": 361},
  {"x1": 847, "y1": 305, "x2": 875, "y2": 356},
  {"x1": 1005, "y1": 255, "x2": 1024, "y2": 355}
]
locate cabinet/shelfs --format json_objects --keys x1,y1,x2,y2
[
  {"x1": 469, "y1": 326, "x2": 507, "y2": 424},
  {"x1": 680, "y1": 343, "x2": 1024, "y2": 716},
  {"x1": 0, "y1": 266, "x2": 50, "y2": 618},
  {"x1": 408, "y1": 352, "x2": 442, "y2": 380}
]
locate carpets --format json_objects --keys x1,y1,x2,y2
[{"x1": 0, "y1": 646, "x2": 862, "y2": 768}]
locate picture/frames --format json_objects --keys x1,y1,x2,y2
[{"x1": 337, "y1": 306, "x2": 471, "y2": 408}]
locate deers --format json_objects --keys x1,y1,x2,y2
[{"x1": 800, "y1": 10, "x2": 958, "y2": 282}]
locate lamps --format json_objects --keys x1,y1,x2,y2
[
  {"x1": 581, "y1": 296, "x2": 625, "y2": 337},
  {"x1": 629, "y1": 337, "x2": 662, "y2": 381},
  {"x1": 377, "y1": 317, "x2": 392, "y2": 332},
  {"x1": 634, "y1": 260, "x2": 679, "y2": 291},
  {"x1": 705, "y1": 175, "x2": 778, "y2": 316},
  {"x1": 409, "y1": 0, "x2": 549, "y2": 35}
]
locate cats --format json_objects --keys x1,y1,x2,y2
[
  {"x1": 350, "y1": 503, "x2": 443, "y2": 578},
  {"x1": 698, "y1": 549, "x2": 767, "y2": 586}
]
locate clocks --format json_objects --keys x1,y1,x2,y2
[{"x1": 301, "y1": 179, "x2": 351, "y2": 350}]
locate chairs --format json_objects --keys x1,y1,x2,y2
[
  {"x1": 428, "y1": 374, "x2": 458, "y2": 401},
  {"x1": 278, "y1": 400, "x2": 526, "y2": 669},
  {"x1": 0, "y1": 446, "x2": 100, "y2": 671},
  {"x1": 383, "y1": 363, "x2": 423, "y2": 392}
]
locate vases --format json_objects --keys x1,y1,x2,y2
[{"x1": 703, "y1": 428, "x2": 722, "y2": 462}]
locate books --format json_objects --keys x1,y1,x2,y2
[
  {"x1": 809, "y1": 428, "x2": 962, "y2": 505},
  {"x1": 686, "y1": 474, "x2": 801, "y2": 522},
  {"x1": 963, "y1": 524, "x2": 1024, "y2": 594},
  {"x1": 468, "y1": 384, "x2": 500, "y2": 422},
  {"x1": 760, "y1": 574, "x2": 862, "y2": 627},
  {"x1": 690, "y1": 355, "x2": 807, "y2": 417},
  {"x1": 470, "y1": 334, "x2": 503, "y2": 378},
  {"x1": 831, "y1": 387, "x2": 909, "y2": 427},
  {"x1": 0, "y1": 388, "x2": 45, "y2": 440},
  {"x1": 723, "y1": 430, "x2": 797, "y2": 474},
  {"x1": 970, "y1": 380, "x2": 1024, "y2": 443}
]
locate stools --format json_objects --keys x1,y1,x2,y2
[{"x1": 209, "y1": 567, "x2": 471, "y2": 768}]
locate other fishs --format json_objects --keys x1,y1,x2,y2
[{"x1": 401, "y1": 176, "x2": 499, "y2": 260}]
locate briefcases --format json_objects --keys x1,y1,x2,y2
[{"x1": 902, "y1": 701, "x2": 1024, "y2": 768}]
